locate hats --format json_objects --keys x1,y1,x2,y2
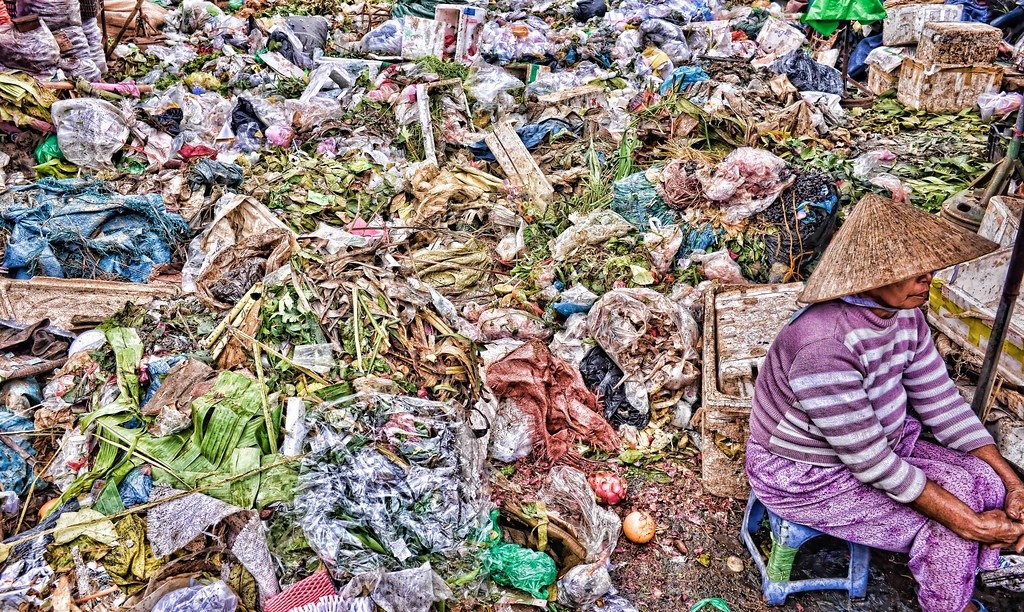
[{"x1": 798, "y1": 191, "x2": 1000, "y2": 301}]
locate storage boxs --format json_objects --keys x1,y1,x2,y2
[
  {"x1": 701, "y1": 283, "x2": 806, "y2": 499},
  {"x1": 868, "y1": 0, "x2": 1004, "y2": 112},
  {"x1": 927, "y1": 195, "x2": 1024, "y2": 387}
]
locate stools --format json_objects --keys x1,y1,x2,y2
[{"x1": 739, "y1": 486, "x2": 870, "y2": 606}]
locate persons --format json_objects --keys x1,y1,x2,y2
[{"x1": 746, "y1": 193, "x2": 1024, "y2": 612}]
